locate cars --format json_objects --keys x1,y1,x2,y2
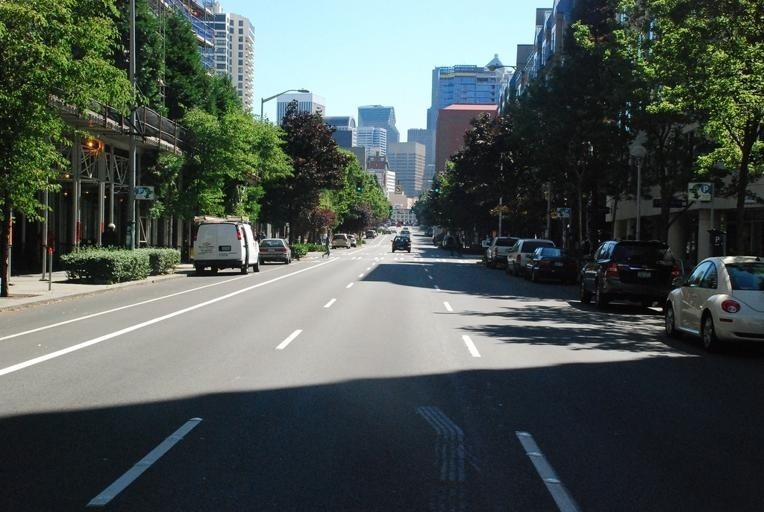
[
  {"x1": 577, "y1": 239, "x2": 686, "y2": 310},
  {"x1": 331, "y1": 233, "x2": 357, "y2": 249},
  {"x1": 366, "y1": 229, "x2": 377, "y2": 238},
  {"x1": 390, "y1": 226, "x2": 412, "y2": 253},
  {"x1": 484, "y1": 236, "x2": 576, "y2": 284},
  {"x1": 258, "y1": 238, "x2": 292, "y2": 264},
  {"x1": 663, "y1": 255, "x2": 764, "y2": 347}
]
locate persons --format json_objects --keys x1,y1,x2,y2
[{"x1": 102, "y1": 223, "x2": 117, "y2": 247}]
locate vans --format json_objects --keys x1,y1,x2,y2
[{"x1": 192, "y1": 221, "x2": 261, "y2": 275}]
